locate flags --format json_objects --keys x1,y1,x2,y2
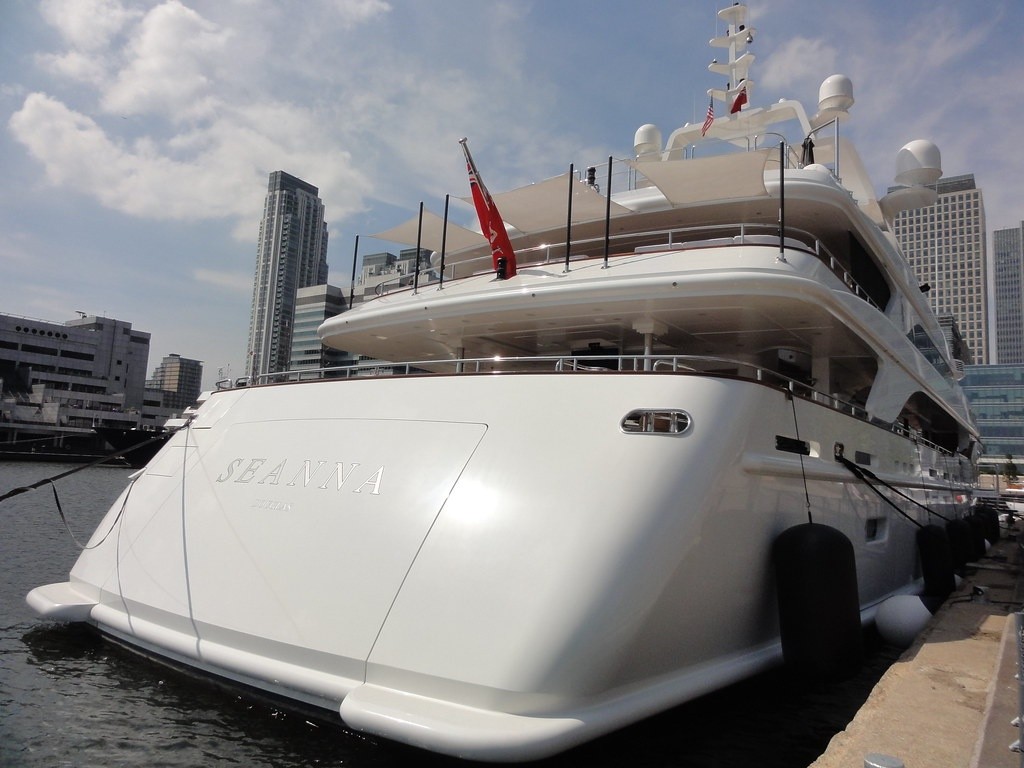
[
  {"x1": 731, "y1": 87, "x2": 747, "y2": 114},
  {"x1": 465, "y1": 151, "x2": 516, "y2": 279},
  {"x1": 702, "y1": 96, "x2": 713, "y2": 136}
]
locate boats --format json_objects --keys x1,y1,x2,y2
[
  {"x1": 0, "y1": 443, "x2": 65, "y2": 463},
  {"x1": 91, "y1": 391, "x2": 216, "y2": 470}
]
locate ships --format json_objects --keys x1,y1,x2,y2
[{"x1": 26, "y1": 1, "x2": 1001, "y2": 765}]
